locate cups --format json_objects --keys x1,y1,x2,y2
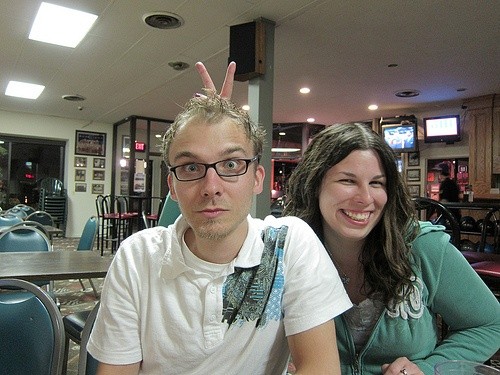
[{"x1": 433, "y1": 360, "x2": 500, "y2": 375}]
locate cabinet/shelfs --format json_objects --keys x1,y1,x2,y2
[{"x1": 436, "y1": 201, "x2": 500, "y2": 237}]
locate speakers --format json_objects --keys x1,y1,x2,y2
[{"x1": 229, "y1": 21, "x2": 266, "y2": 81}]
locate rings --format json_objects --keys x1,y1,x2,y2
[{"x1": 400, "y1": 369, "x2": 407, "y2": 375}]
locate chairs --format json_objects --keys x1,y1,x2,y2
[{"x1": 0, "y1": 177, "x2": 99, "y2": 375}]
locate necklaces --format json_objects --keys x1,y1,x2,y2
[{"x1": 324, "y1": 241, "x2": 350, "y2": 286}]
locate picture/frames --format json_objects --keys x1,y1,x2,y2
[
  {"x1": 120, "y1": 136, "x2": 148, "y2": 193},
  {"x1": 415, "y1": 207, "x2": 420, "y2": 221},
  {"x1": 91, "y1": 183, "x2": 104, "y2": 194},
  {"x1": 74, "y1": 157, "x2": 88, "y2": 167},
  {"x1": 406, "y1": 169, "x2": 421, "y2": 182},
  {"x1": 407, "y1": 184, "x2": 421, "y2": 197},
  {"x1": 75, "y1": 168, "x2": 85, "y2": 180},
  {"x1": 408, "y1": 150, "x2": 420, "y2": 167},
  {"x1": 93, "y1": 158, "x2": 106, "y2": 168},
  {"x1": 75, "y1": 183, "x2": 86, "y2": 193},
  {"x1": 75, "y1": 129, "x2": 106, "y2": 157},
  {"x1": 93, "y1": 170, "x2": 105, "y2": 180}
]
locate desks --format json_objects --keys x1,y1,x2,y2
[
  {"x1": 115, "y1": 193, "x2": 160, "y2": 237},
  {"x1": 0, "y1": 224, "x2": 63, "y2": 250},
  {"x1": 0, "y1": 247, "x2": 111, "y2": 302}
]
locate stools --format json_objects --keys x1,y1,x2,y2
[
  {"x1": 405, "y1": 195, "x2": 500, "y2": 368},
  {"x1": 96, "y1": 194, "x2": 166, "y2": 255}
]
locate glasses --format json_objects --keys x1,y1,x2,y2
[{"x1": 170, "y1": 154, "x2": 259, "y2": 181}]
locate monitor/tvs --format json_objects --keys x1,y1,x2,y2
[
  {"x1": 381, "y1": 124, "x2": 417, "y2": 152},
  {"x1": 423, "y1": 115, "x2": 460, "y2": 142}
]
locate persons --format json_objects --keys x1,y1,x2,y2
[
  {"x1": 159, "y1": 61, "x2": 500, "y2": 375},
  {"x1": 429, "y1": 163, "x2": 462, "y2": 224},
  {"x1": 86, "y1": 95, "x2": 352, "y2": 375}
]
